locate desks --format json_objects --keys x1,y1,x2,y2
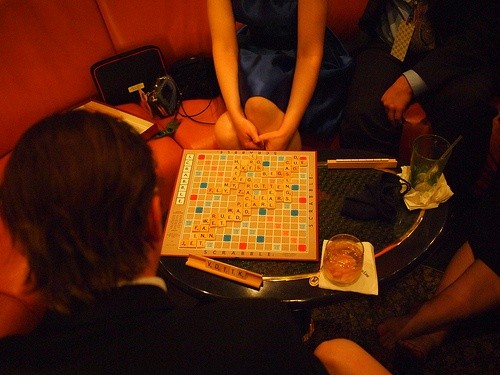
[{"x1": 158, "y1": 150, "x2": 450, "y2": 342}]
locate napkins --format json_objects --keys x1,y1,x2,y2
[
  {"x1": 319, "y1": 234, "x2": 379, "y2": 297},
  {"x1": 397, "y1": 161, "x2": 454, "y2": 212}
]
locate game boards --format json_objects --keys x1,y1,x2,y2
[{"x1": 159, "y1": 148, "x2": 319, "y2": 263}]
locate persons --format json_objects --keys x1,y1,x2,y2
[
  {"x1": 375, "y1": 167, "x2": 500, "y2": 360},
  {"x1": 341, "y1": 0, "x2": 500, "y2": 231},
  {"x1": 0, "y1": 110, "x2": 329, "y2": 375},
  {"x1": 206, "y1": 0, "x2": 353, "y2": 151}
]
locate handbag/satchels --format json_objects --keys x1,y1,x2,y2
[
  {"x1": 406, "y1": 0, "x2": 443, "y2": 53},
  {"x1": 90, "y1": 45, "x2": 168, "y2": 107},
  {"x1": 147, "y1": 73, "x2": 181, "y2": 118},
  {"x1": 168, "y1": 55, "x2": 220, "y2": 99}
]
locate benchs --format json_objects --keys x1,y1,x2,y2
[{"x1": 0, "y1": 0, "x2": 368, "y2": 336}]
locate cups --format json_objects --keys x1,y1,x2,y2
[
  {"x1": 409, "y1": 134, "x2": 452, "y2": 194},
  {"x1": 324, "y1": 234, "x2": 364, "y2": 286}
]
